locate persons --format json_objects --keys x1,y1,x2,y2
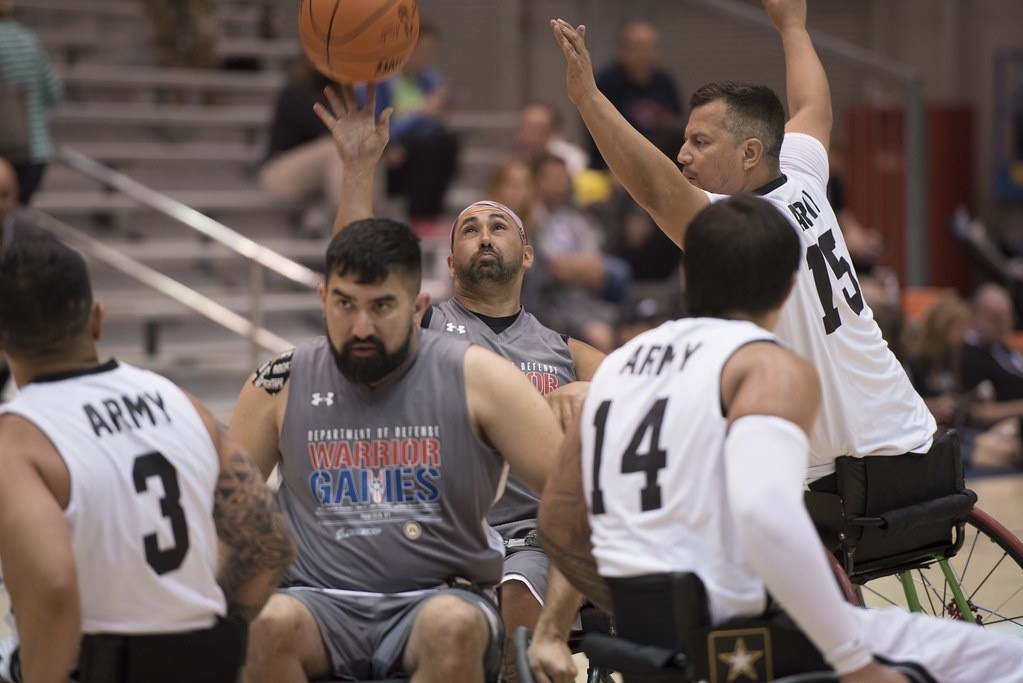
[
  {"x1": 0, "y1": 235, "x2": 296, "y2": 683},
  {"x1": 0, "y1": 77, "x2": 32, "y2": 394},
  {"x1": 224, "y1": 219, "x2": 585, "y2": 683},
  {"x1": 0, "y1": 0, "x2": 63, "y2": 202},
  {"x1": 537, "y1": 194, "x2": 1022, "y2": 683},
  {"x1": 550, "y1": 0, "x2": 938, "y2": 492},
  {"x1": 492, "y1": 24, "x2": 690, "y2": 351},
  {"x1": 832, "y1": 148, "x2": 1023, "y2": 476},
  {"x1": 311, "y1": 74, "x2": 609, "y2": 682},
  {"x1": 359, "y1": 24, "x2": 463, "y2": 236},
  {"x1": 258, "y1": 49, "x2": 348, "y2": 237}
]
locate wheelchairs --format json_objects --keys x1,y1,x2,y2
[
  {"x1": 499, "y1": 626, "x2": 539, "y2": 683},
  {"x1": 803, "y1": 424, "x2": 1023, "y2": 637},
  {"x1": 578, "y1": 567, "x2": 941, "y2": 683}
]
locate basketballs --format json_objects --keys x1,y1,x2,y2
[{"x1": 297, "y1": 1, "x2": 422, "y2": 89}]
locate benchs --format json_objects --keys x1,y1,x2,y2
[{"x1": 10, "y1": 1, "x2": 529, "y2": 376}]
